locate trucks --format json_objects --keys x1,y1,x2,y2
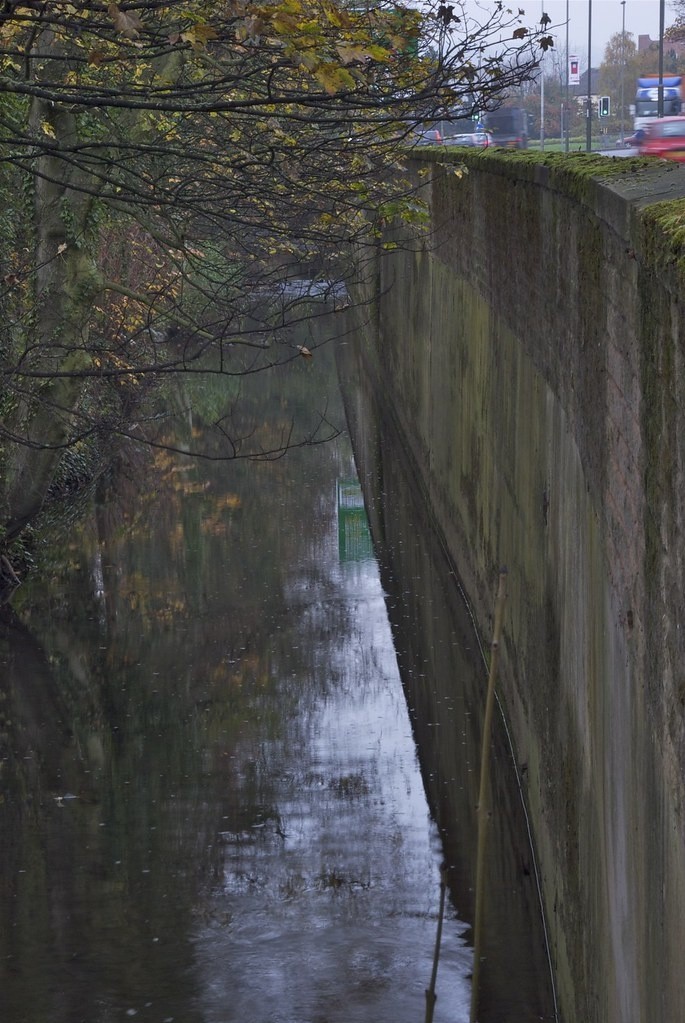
[{"x1": 629, "y1": 73, "x2": 685, "y2": 135}]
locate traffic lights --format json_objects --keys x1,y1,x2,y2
[{"x1": 601, "y1": 98, "x2": 611, "y2": 117}]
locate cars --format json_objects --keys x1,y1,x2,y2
[
  {"x1": 411, "y1": 129, "x2": 443, "y2": 149},
  {"x1": 615, "y1": 129, "x2": 648, "y2": 150},
  {"x1": 439, "y1": 131, "x2": 493, "y2": 150},
  {"x1": 638, "y1": 116, "x2": 685, "y2": 164}
]
